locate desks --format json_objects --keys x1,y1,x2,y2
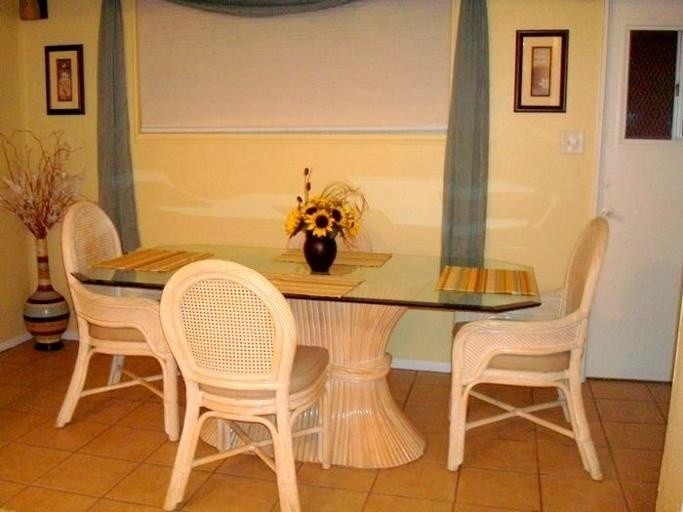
[{"x1": 70, "y1": 244, "x2": 541, "y2": 470}]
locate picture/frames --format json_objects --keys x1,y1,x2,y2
[
  {"x1": 44, "y1": 44, "x2": 85, "y2": 116},
  {"x1": 513, "y1": 28, "x2": 570, "y2": 112}
]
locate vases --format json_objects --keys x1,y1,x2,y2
[
  {"x1": 303, "y1": 230, "x2": 337, "y2": 276},
  {"x1": 23, "y1": 239, "x2": 69, "y2": 352}
]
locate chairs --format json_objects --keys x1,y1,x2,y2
[
  {"x1": 158, "y1": 257, "x2": 332, "y2": 512},
  {"x1": 53, "y1": 199, "x2": 181, "y2": 442},
  {"x1": 448, "y1": 217, "x2": 610, "y2": 481}
]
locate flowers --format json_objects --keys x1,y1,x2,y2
[
  {"x1": 282, "y1": 164, "x2": 371, "y2": 252},
  {"x1": 0, "y1": 125, "x2": 88, "y2": 241}
]
